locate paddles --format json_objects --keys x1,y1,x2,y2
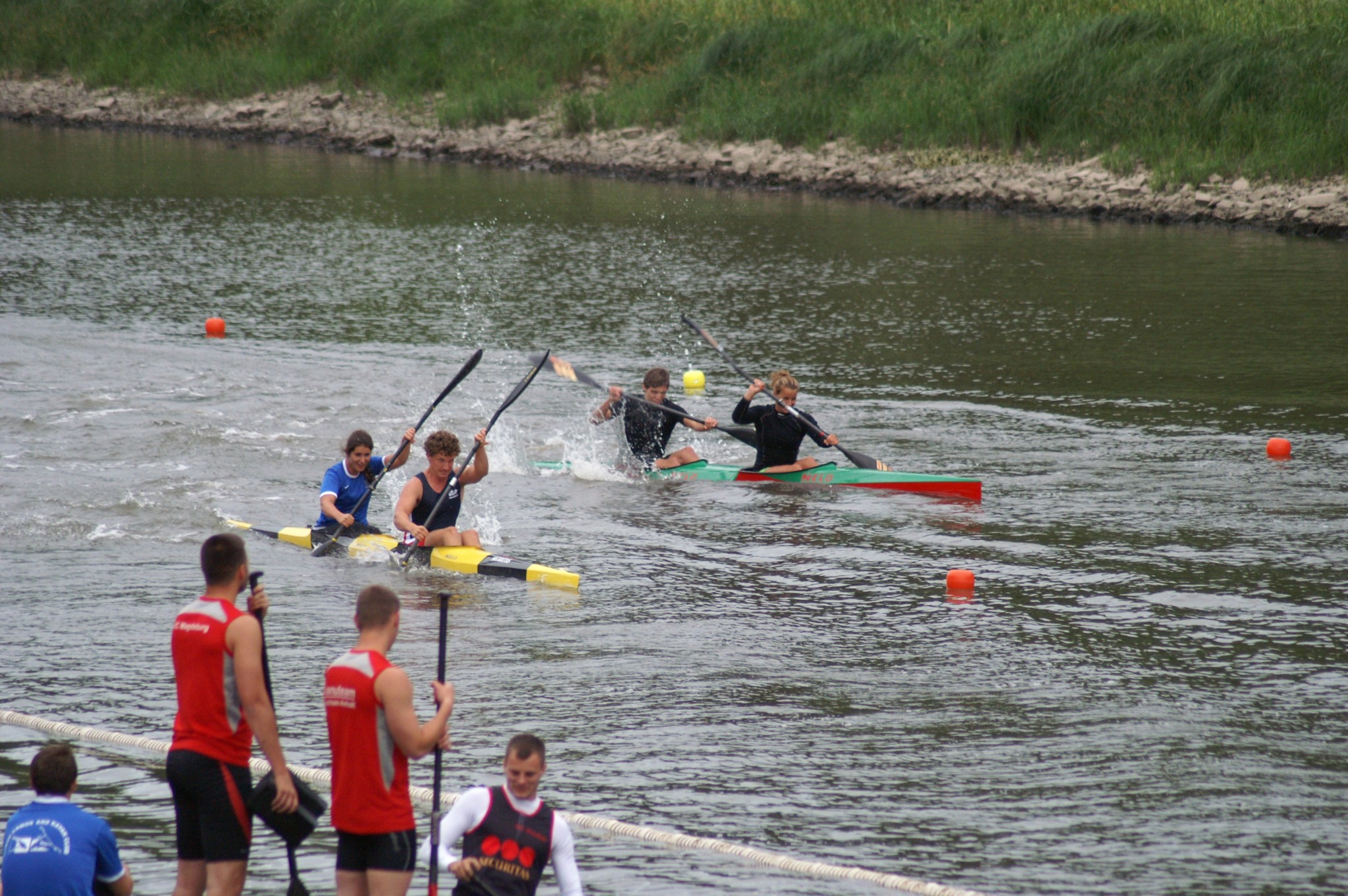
[
  {"x1": 411, "y1": 589, "x2": 454, "y2": 896},
  {"x1": 401, "y1": 350, "x2": 552, "y2": 568},
  {"x1": 246, "y1": 568, "x2": 310, "y2": 896},
  {"x1": 526, "y1": 353, "x2": 759, "y2": 448},
  {"x1": 678, "y1": 313, "x2": 892, "y2": 473},
  {"x1": 310, "y1": 349, "x2": 481, "y2": 558}
]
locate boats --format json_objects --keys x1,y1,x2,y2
[
  {"x1": 227, "y1": 517, "x2": 579, "y2": 590},
  {"x1": 536, "y1": 460, "x2": 982, "y2": 503}
]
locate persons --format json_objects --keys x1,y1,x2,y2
[
  {"x1": 589, "y1": 367, "x2": 717, "y2": 470},
  {"x1": 324, "y1": 586, "x2": 455, "y2": 896},
  {"x1": 417, "y1": 734, "x2": 585, "y2": 896},
  {"x1": 394, "y1": 428, "x2": 489, "y2": 548},
  {"x1": 312, "y1": 428, "x2": 416, "y2": 539},
  {"x1": 732, "y1": 369, "x2": 840, "y2": 473},
  {"x1": 0, "y1": 745, "x2": 133, "y2": 896},
  {"x1": 166, "y1": 532, "x2": 300, "y2": 896}
]
